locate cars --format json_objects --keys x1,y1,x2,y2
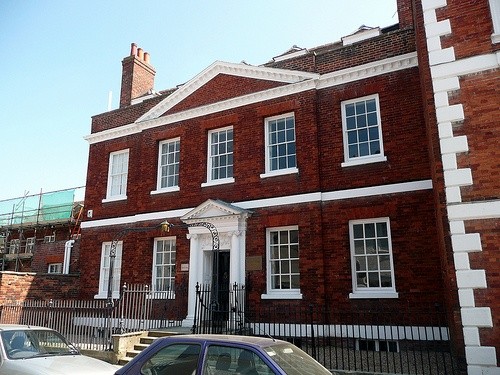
[
  {"x1": 0, "y1": 323, "x2": 124, "y2": 375},
  {"x1": 113, "y1": 334, "x2": 332, "y2": 375}
]
